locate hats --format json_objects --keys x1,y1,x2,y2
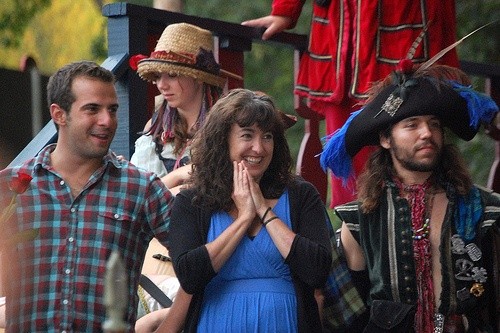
[
  {"x1": 319, "y1": 17, "x2": 500, "y2": 188},
  {"x1": 129, "y1": 24, "x2": 228, "y2": 87}
]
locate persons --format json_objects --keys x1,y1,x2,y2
[
  {"x1": 129, "y1": 23, "x2": 243, "y2": 313},
  {"x1": 322, "y1": 19, "x2": 500, "y2": 333},
  {"x1": 242, "y1": 0, "x2": 460, "y2": 209},
  {"x1": 0, "y1": 60, "x2": 192, "y2": 333},
  {"x1": 136, "y1": 90, "x2": 366, "y2": 333}
]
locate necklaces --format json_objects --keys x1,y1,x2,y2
[
  {"x1": 414, "y1": 219, "x2": 430, "y2": 232},
  {"x1": 412, "y1": 226, "x2": 430, "y2": 239}
]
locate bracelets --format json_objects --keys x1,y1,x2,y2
[
  {"x1": 261, "y1": 207, "x2": 272, "y2": 222},
  {"x1": 264, "y1": 216, "x2": 279, "y2": 226}
]
